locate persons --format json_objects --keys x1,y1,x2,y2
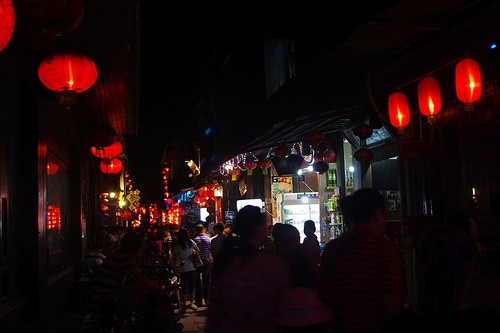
[
  {"x1": 48, "y1": 223, "x2": 212, "y2": 333},
  {"x1": 318, "y1": 188, "x2": 500, "y2": 333},
  {"x1": 206, "y1": 206, "x2": 334, "y2": 333}
]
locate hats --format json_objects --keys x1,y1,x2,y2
[{"x1": 271, "y1": 286, "x2": 330, "y2": 327}]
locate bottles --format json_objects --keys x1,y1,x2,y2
[
  {"x1": 328, "y1": 170, "x2": 337, "y2": 188},
  {"x1": 324, "y1": 194, "x2": 343, "y2": 239}
]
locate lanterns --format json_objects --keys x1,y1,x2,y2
[
  {"x1": 274, "y1": 145, "x2": 290, "y2": 159},
  {"x1": 304, "y1": 131, "x2": 336, "y2": 174},
  {"x1": 353, "y1": 124, "x2": 374, "y2": 163},
  {"x1": 417, "y1": 77, "x2": 442, "y2": 124},
  {"x1": 86, "y1": 123, "x2": 124, "y2": 175},
  {"x1": 195, "y1": 182, "x2": 215, "y2": 203},
  {"x1": 455, "y1": 58, "x2": 483, "y2": 103},
  {"x1": 48, "y1": 163, "x2": 58, "y2": 174},
  {"x1": 38, "y1": 49, "x2": 98, "y2": 105},
  {"x1": 0, "y1": 0, "x2": 14, "y2": 51},
  {"x1": 229, "y1": 158, "x2": 272, "y2": 181},
  {"x1": 101, "y1": 192, "x2": 158, "y2": 227},
  {"x1": 388, "y1": 92, "x2": 411, "y2": 134}
]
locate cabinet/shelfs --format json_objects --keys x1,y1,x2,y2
[{"x1": 317, "y1": 163, "x2": 355, "y2": 243}]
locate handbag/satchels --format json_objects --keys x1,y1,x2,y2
[{"x1": 192, "y1": 253, "x2": 205, "y2": 269}]
